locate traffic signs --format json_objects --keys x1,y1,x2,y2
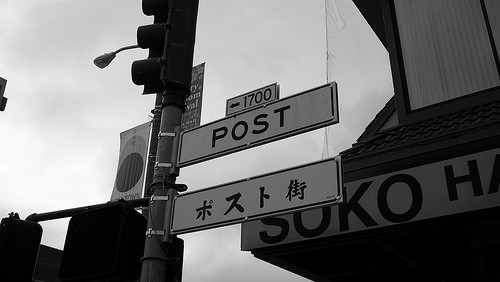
[{"x1": 168, "y1": 154, "x2": 341, "y2": 233}]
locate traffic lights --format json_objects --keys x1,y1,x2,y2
[{"x1": 130, "y1": 0, "x2": 201, "y2": 106}]
